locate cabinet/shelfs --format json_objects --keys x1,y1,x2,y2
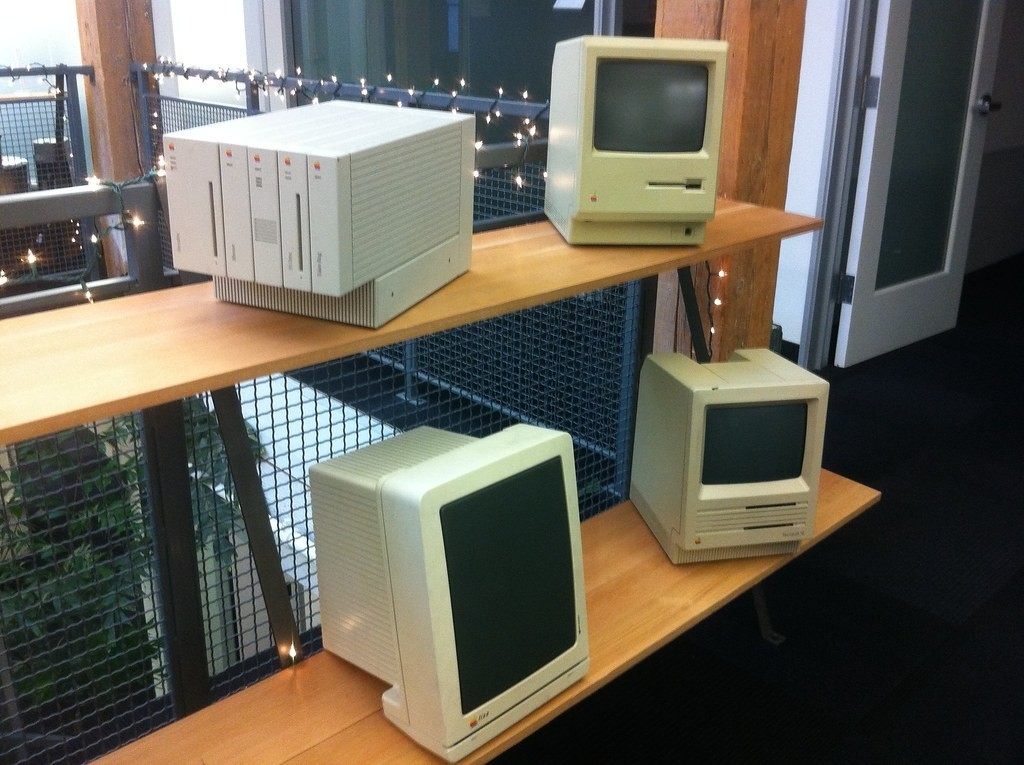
[{"x1": 0, "y1": 200, "x2": 882, "y2": 765}]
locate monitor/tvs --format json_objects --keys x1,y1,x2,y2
[
  {"x1": 308, "y1": 423, "x2": 589, "y2": 764},
  {"x1": 630, "y1": 347, "x2": 830, "y2": 564},
  {"x1": 545, "y1": 34, "x2": 729, "y2": 244}
]
